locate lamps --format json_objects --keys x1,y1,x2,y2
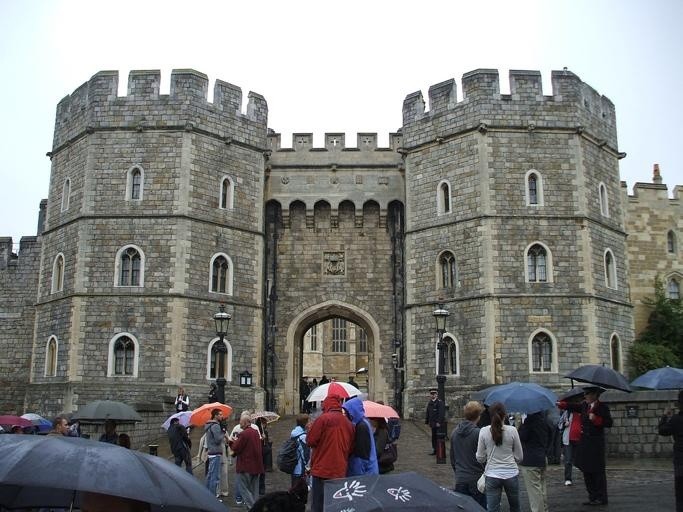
[{"x1": 240, "y1": 371, "x2": 252, "y2": 386}]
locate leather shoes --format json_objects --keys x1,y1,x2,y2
[{"x1": 582, "y1": 498, "x2": 608, "y2": 507}]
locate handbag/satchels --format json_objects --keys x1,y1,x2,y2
[
  {"x1": 377, "y1": 423, "x2": 398, "y2": 467},
  {"x1": 476, "y1": 471, "x2": 488, "y2": 497}
]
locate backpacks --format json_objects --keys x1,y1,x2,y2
[
  {"x1": 385, "y1": 416, "x2": 402, "y2": 443},
  {"x1": 276, "y1": 431, "x2": 308, "y2": 476}
]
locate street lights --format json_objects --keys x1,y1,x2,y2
[
  {"x1": 211, "y1": 304, "x2": 230, "y2": 432},
  {"x1": 431, "y1": 297, "x2": 449, "y2": 463}
]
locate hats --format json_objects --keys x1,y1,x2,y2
[
  {"x1": 428, "y1": 388, "x2": 440, "y2": 395},
  {"x1": 582, "y1": 385, "x2": 601, "y2": 396}
]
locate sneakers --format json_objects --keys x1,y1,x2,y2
[
  {"x1": 564, "y1": 480, "x2": 573, "y2": 486},
  {"x1": 215, "y1": 492, "x2": 229, "y2": 504},
  {"x1": 235, "y1": 496, "x2": 243, "y2": 505}
]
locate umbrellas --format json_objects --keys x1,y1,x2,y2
[
  {"x1": 0, "y1": 412, "x2": 32, "y2": 427},
  {"x1": 0, "y1": 433, "x2": 228, "y2": 512},
  {"x1": 558, "y1": 383, "x2": 607, "y2": 402},
  {"x1": 471, "y1": 381, "x2": 557, "y2": 417},
  {"x1": 630, "y1": 365, "x2": 683, "y2": 408},
  {"x1": 322, "y1": 469, "x2": 487, "y2": 512},
  {"x1": 21, "y1": 413, "x2": 52, "y2": 431},
  {"x1": 562, "y1": 361, "x2": 633, "y2": 394},
  {"x1": 71, "y1": 400, "x2": 144, "y2": 440},
  {"x1": 56, "y1": 410, "x2": 78, "y2": 426}
]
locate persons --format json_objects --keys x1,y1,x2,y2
[
  {"x1": 119, "y1": 433, "x2": 130, "y2": 449},
  {"x1": 79, "y1": 492, "x2": 151, "y2": 512},
  {"x1": 166, "y1": 374, "x2": 399, "y2": 512},
  {"x1": 449, "y1": 399, "x2": 573, "y2": 512},
  {"x1": 98, "y1": 420, "x2": 118, "y2": 445},
  {"x1": 658, "y1": 389, "x2": 683, "y2": 512},
  {"x1": 48, "y1": 417, "x2": 69, "y2": 436},
  {"x1": 555, "y1": 386, "x2": 613, "y2": 505},
  {"x1": 425, "y1": 388, "x2": 444, "y2": 455}
]
